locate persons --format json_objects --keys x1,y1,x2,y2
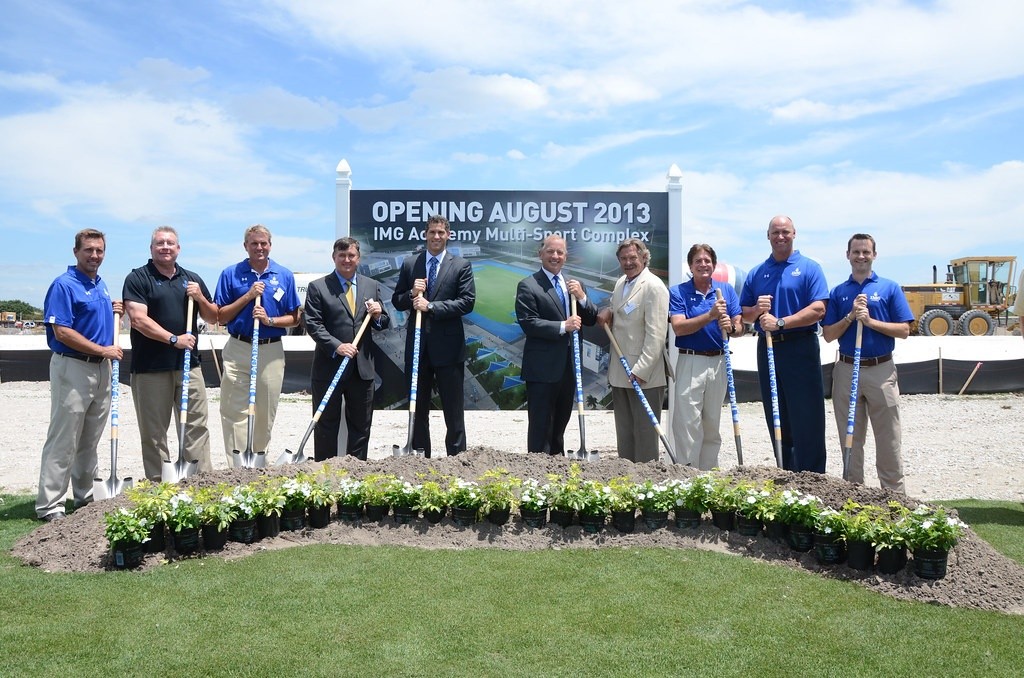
[
  {"x1": 213, "y1": 225, "x2": 302, "y2": 467},
  {"x1": 122, "y1": 226, "x2": 221, "y2": 481},
  {"x1": 739, "y1": 216, "x2": 831, "y2": 475},
  {"x1": 668, "y1": 244, "x2": 746, "y2": 471},
  {"x1": 596, "y1": 238, "x2": 669, "y2": 464},
  {"x1": 36, "y1": 228, "x2": 126, "y2": 520},
  {"x1": 390, "y1": 215, "x2": 476, "y2": 459},
  {"x1": 514, "y1": 235, "x2": 599, "y2": 456},
  {"x1": 817, "y1": 234, "x2": 915, "y2": 495},
  {"x1": 303, "y1": 237, "x2": 389, "y2": 462}
]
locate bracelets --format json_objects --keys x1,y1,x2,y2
[
  {"x1": 845, "y1": 314, "x2": 853, "y2": 324},
  {"x1": 268, "y1": 317, "x2": 274, "y2": 326}
]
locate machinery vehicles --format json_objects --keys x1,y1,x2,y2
[{"x1": 901, "y1": 257, "x2": 1018, "y2": 336}]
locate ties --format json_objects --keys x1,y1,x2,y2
[
  {"x1": 427, "y1": 257, "x2": 439, "y2": 298},
  {"x1": 554, "y1": 276, "x2": 565, "y2": 306},
  {"x1": 345, "y1": 280, "x2": 356, "y2": 317}
]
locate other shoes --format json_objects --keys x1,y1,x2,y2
[{"x1": 42, "y1": 511, "x2": 66, "y2": 522}]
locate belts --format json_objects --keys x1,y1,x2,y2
[
  {"x1": 58, "y1": 351, "x2": 104, "y2": 363},
  {"x1": 232, "y1": 333, "x2": 281, "y2": 344},
  {"x1": 840, "y1": 354, "x2": 893, "y2": 367},
  {"x1": 679, "y1": 348, "x2": 724, "y2": 357},
  {"x1": 772, "y1": 330, "x2": 814, "y2": 341}
]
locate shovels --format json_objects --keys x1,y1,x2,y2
[
  {"x1": 161, "y1": 296, "x2": 198, "y2": 486},
  {"x1": 93, "y1": 313, "x2": 133, "y2": 501},
  {"x1": 232, "y1": 293, "x2": 265, "y2": 469},
  {"x1": 567, "y1": 293, "x2": 599, "y2": 461},
  {"x1": 393, "y1": 289, "x2": 425, "y2": 458},
  {"x1": 274, "y1": 312, "x2": 373, "y2": 468}
]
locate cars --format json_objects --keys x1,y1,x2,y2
[{"x1": 23, "y1": 322, "x2": 35, "y2": 328}]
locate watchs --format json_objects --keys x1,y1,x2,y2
[
  {"x1": 169, "y1": 335, "x2": 178, "y2": 346},
  {"x1": 727, "y1": 323, "x2": 736, "y2": 335},
  {"x1": 776, "y1": 318, "x2": 785, "y2": 331},
  {"x1": 427, "y1": 302, "x2": 434, "y2": 313}
]
